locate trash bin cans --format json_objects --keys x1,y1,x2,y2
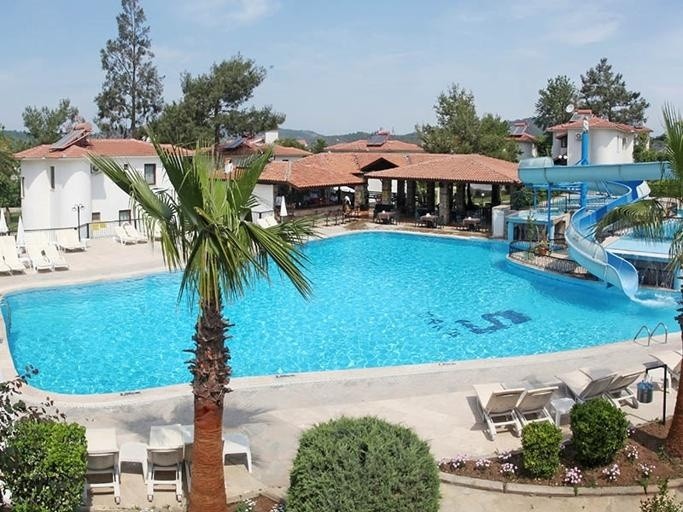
[{"x1": 637, "y1": 381, "x2": 653, "y2": 403}]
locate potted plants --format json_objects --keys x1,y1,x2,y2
[{"x1": 533, "y1": 239, "x2": 552, "y2": 258}]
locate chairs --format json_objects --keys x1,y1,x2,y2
[
  {"x1": 258, "y1": 215, "x2": 283, "y2": 233},
  {"x1": 114, "y1": 217, "x2": 149, "y2": 248},
  {"x1": 2, "y1": 226, "x2": 87, "y2": 275},
  {"x1": 76, "y1": 418, "x2": 252, "y2": 506},
  {"x1": 473, "y1": 346, "x2": 683, "y2": 440}
]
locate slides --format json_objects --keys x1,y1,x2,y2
[{"x1": 518, "y1": 157, "x2": 683, "y2": 309}]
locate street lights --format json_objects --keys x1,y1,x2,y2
[{"x1": 72, "y1": 204, "x2": 85, "y2": 243}]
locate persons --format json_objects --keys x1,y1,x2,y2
[{"x1": 275, "y1": 192, "x2": 351, "y2": 217}]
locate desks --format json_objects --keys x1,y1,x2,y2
[{"x1": 376, "y1": 209, "x2": 482, "y2": 232}]
